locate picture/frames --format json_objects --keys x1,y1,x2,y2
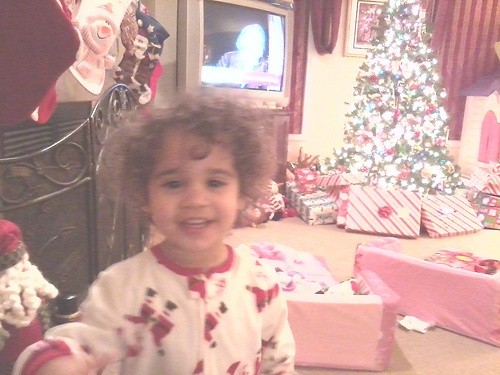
[{"x1": 344, "y1": 0, "x2": 388, "y2": 59}]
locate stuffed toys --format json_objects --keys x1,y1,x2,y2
[
  {"x1": 69, "y1": 0, "x2": 171, "y2": 104},
  {"x1": 0, "y1": 219, "x2": 60, "y2": 375}
]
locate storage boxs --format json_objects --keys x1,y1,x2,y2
[{"x1": 285, "y1": 146, "x2": 500, "y2": 240}]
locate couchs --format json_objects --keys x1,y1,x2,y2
[
  {"x1": 352, "y1": 238, "x2": 499, "y2": 348},
  {"x1": 243, "y1": 244, "x2": 402, "y2": 372}
]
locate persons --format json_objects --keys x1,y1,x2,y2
[{"x1": 12, "y1": 87, "x2": 298, "y2": 374}]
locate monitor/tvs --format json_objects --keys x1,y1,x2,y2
[{"x1": 177, "y1": 0, "x2": 294, "y2": 112}]
